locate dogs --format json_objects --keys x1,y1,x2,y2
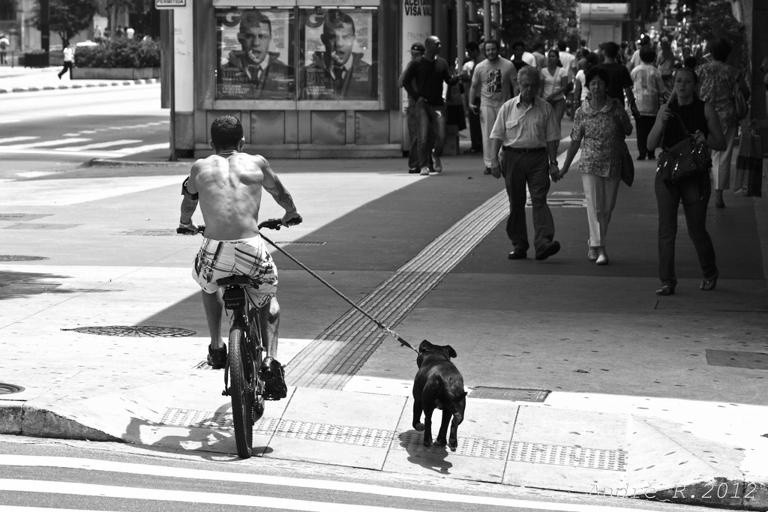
[{"x1": 411, "y1": 340, "x2": 467, "y2": 452}]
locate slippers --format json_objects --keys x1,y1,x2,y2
[
  {"x1": 657, "y1": 284, "x2": 675, "y2": 295},
  {"x1": 701, "y1": 269, "x2": 718, "y2": 290}
]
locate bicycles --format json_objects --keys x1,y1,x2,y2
[{"x1": 175, "y1": 217, "x2": 301, "y2": 456}]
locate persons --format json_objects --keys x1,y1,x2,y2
[
  {"x1": 306, "y1": 13, "x2": 374, "y2": 100},
  {"x1": 178, "y1": 115, "x2": 300, "y2": 398},
  {"x1": 58, "y1": 43, "x2": 74, "y2": 81},
  {"x1": 95, "y1": 24, "x2": 153, "y2": 43},
  {"x1": 0, "y1": 35, "x2": 9, "y2": 64},
  {"x1": 221, "y1": 10, "x2": 295, "y2": 100}
]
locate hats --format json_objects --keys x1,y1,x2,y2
[{"x1": 408, "y1": 42, "x2": 424, "y2": 54}]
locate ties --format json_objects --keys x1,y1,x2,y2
[
  {"x1": 333, "y1": 66, "x2": 344, "y2": 93},
  {"x1": 249, "y1": 65, "x2": 260, "y2": 86}
]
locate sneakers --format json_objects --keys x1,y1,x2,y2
[
  {"x1": 588, "y1": 239, "x2": 608, "y2": 265},
  {"x1": 508, "y1": 247, "x2": 527, "y2": 259},
  {"x1": 260, "y1": 356, "x2": 286, "y2": 400},
  {"x1": 408, "y1": 156, "x2": 441, "y2": 175},
  {"x1": 535, "y1": 241, "x2": 560, "y2": 260},
  {"x1": 208, "y1": 343, "x2": 227, "y2": 368}
]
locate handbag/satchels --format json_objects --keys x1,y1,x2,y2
[
  {"x1": 655, "y1": 142, "x2": 705, "y2": 185},
  {"x1": 622, "y1": 145, "x2": 634, "y2": 187}
]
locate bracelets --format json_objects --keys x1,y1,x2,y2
[{"x1": 179, "y1": 222, "x2": 192, "y2": 228}]
